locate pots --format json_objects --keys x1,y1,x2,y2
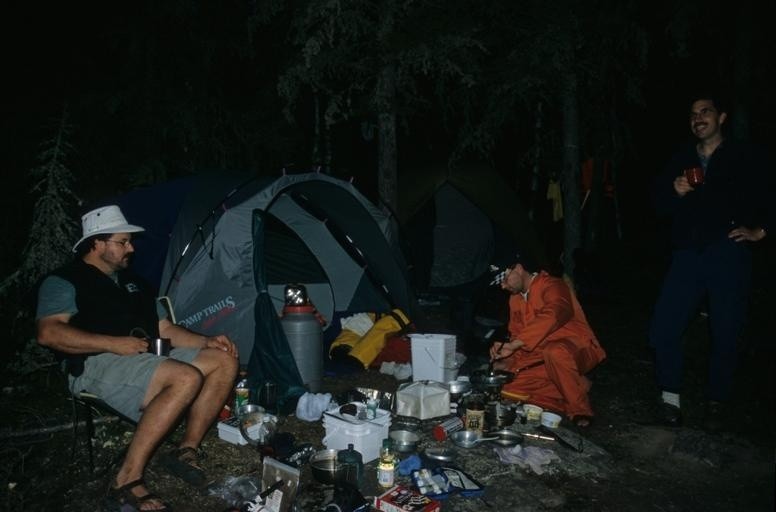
[
  {"x1": 309, "y1": 449, "x2": 346, "y2": 484},
  {"x1": 485, "y1": 401, "x2": 517, "y2": 427},
  {"x1": 450, "y1": 430, "x2": 500, "y2": 447},
  {"x1": 472, "y1": 359, "x2": 547, "y2": 388}
]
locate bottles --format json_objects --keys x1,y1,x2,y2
[
  {"x1": 378, "y1": 448, "x2": 395, "y2": 487},
  {"x1": 367, "y1": 398, "x2": 377, "y2": 420},
  {"x1": 465, "y1": 397, "x2": 485, "y2": 432},
  {"x1": 219, "y1": 369, "x2": 250, "y2": 420},
  {"x1": 358, "y1": 407, "x2": 366, "y2": 420}
]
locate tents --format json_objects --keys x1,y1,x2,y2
[
  {"x1": 87, "y1": 170, "x2": 428, "y2": 411},
  {"x1": 394, "y1": 160, "x2": 547, "y2": 309}
]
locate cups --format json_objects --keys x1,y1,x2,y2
[
  {"x1": 258, "y1": 381, "x2": 276, "y2": 412},
  {"x1": 146, "y1": 337, "x2": 171, "y2": 357},
  {"x1": 337, "y1": 444, "x2": 365, "y2": 492},
  {"x1": 687, "y1": 166, "x2": 703, "y2": 187}
]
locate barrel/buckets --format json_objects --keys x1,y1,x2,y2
[{"x1": 406, "y1": 332, "x2": 460, "y2": 387}]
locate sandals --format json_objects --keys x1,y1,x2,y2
[
  {"x1": 166, "y1": 447, "x2": 205, "y2": 486},
  {"x1": 572, "y1": 414, "x2": 592, "y2": 427},
  {"x1": 108, "y1": 478, "x2": 165, "y2": 512}
]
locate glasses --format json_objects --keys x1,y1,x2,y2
[{"x1": 101, "y1": 238, "x2": 137, "y2": 247}]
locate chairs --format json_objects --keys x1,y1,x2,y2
[{"x1": 38, "y1": 320, "x2": 184, "y2": 468}]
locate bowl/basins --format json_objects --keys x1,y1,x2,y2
[
  {"x1": 524, "y1": 404, "x2": 562, "y2": 430},
  {"x1": 339, "y1": 403, "x2": 357, "y2": 417},
  {"x1": 390, "y1": 429, "x2": 421, "y2": 452},
  {"x1": 233, "y1": 404, "x2": 265, "y2": 421}
]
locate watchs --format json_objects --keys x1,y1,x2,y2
[{"x1": 758, "y1": 223, "x2": 769, "y2": 233}]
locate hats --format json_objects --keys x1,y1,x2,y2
[
  {"x1": 488, "y1": 244, "x2": 518, "y2": 286},
  {"x1": 72, "y1": 205, "x2": 144, "y2": 252}
]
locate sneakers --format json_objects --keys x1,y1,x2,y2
[{"x1": 642, "y1": 400, "x2": 725, "y2": 431}]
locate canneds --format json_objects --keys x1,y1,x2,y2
[
  {"x1": 465, "y1": 401, "x2": 485, "y2": 432},
  {"x1": 378, "y1": 458, "x2": 395, "y2": 488}
]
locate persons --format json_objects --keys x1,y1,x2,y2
[
  {"x1": 637, "y1": 96, "x2": 776, "y2": 426},
  {"x1": 34, "y1": 204, "x2": 239, "y2": 512},
  {"x1": 485, "y1": 249, "x2": 607, "y2": 429}
]
